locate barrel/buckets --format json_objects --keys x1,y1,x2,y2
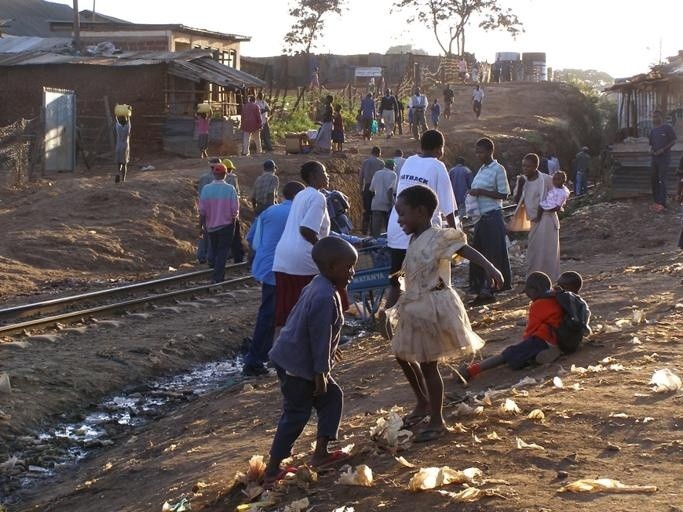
[
  {"x1": 401, "y1": 410, "x2": 431, "y2": 429},
  {"x1": 311, "y1": 451, "x2": 349, "y2": 470},
  {"x1": 413, "y1": 428, "x2": 449, "y2": 442},
  {"x1": 262, "y1": 467, "x2": 297, "y2": 490}
]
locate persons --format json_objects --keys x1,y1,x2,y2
[
  {"x1": 430, "y1": 98, "x2": 441, "y2": 130},
  {"x1": 267, "y1": 160, "x2": 331, "y2": 368},
  {"x1": 448, "y1": 157, "x2": 474, "y2": 216},
  {"x1": 261, "y1": 235, "x2": 359, "y2": 484},
  {"x1": 647, "y1": 109, "x2": 678, "y2": 212},
  {"x1": 196, "y1": 155, "x2": 245, "y2": 291},
  {"x1": 388, "y1": 183, "x2": 505, "y2": 443},
  {"x1": 247, "y1": 159, "x2": 279, "y2": 273},
  {"x1": 358, "y1": 146, "x2": 407, "y2": 237},
  {"x1": 471, "y1": 85, "x2": 484, "y2": 119},
  {"x1": 194, "y1": 106, "x2": 213, "y2": 159},
  {"x1": 442, "y1": 83, "x2": 455, "y2": 120},
  {"x1": 309, "y1": 95, "x2": 345, "y2": 156},
  {"x1": 357, "y1": 87, "x2": 429, "y2": 141},
  {"x1": 240, "y1": 91, "x2": 275, "y2": 157},
  {"x1": 238, "y1": 181, "x2": 306, "y2": 379},
  {"x1": 113, "y1": 103, "x2": 131, "y2": 183},
  {"x1": 460, "y1": 59, "x2": 468, "y2": 85},
  {"x1": 379, "y1": 130, "x2": 459, "y2": 341},
  {"x1": 467, "y1": 137, "x2": 511, "y2": 307},
  {"x1": 456, "y1": 273, "x2": 590, "y2": 386},
  {"x1": 513, "y1": 153, "x2": 570, "y2": 286}
]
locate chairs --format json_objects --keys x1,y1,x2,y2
[
  {"x1": 457, "y1": 362, "x2": 471, "y2": 384},
  {"x1": 243, "y1": 364, "x2": 269, "y2": 376},
  {"x1": 536, "y1": 348, "x2": 561, "y2": 365}
]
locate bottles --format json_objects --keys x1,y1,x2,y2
[
  {"x1": 213, "y1": 164, "x2": 229, "y2": 176},
  {"x1": 208, "y1": 159, "x2": 222, "y2": 166},
  {"x1": 222, "y1": 159, "x2": 236, "y2": 170}
]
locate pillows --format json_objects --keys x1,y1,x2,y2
[{"x1": 264, "y1": 159, "x2": 278, "y2": 171}]
